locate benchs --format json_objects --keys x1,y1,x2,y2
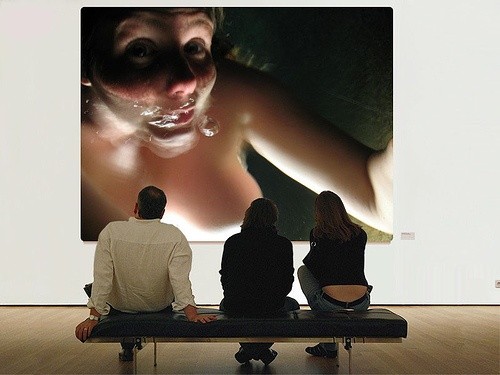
[{"x1": 86, "y1": 308, "x2": 408, "y2": 375}]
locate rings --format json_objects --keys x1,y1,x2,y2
[{"x1": 84, "y1": 328, "x2": 88, "y2": 331}]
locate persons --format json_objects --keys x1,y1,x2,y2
[
  {"x1": 75, "y1": 185, "x2": 217, "y2": 362},
  {"x1": 219, "y1": 198, "x2": 300, "y2": 366},
  {"x1": 298, "y1": 191, "x2": 373, "y2": 358},
  {"x1": 81, "y1": 7, "x2": 394, "y2": 242}
]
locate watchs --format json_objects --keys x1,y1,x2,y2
[{"x1": 89, "y1": 315, "x2": 100, "y2": 321}]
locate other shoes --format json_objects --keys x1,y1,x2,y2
[
  {"x1": 119, "y1": 347, "x2": 133, "y2": 361},
  {"x1": 235, "y1": 347, "x2": 253, "y2": 363},
  {"x1": 261, "y1": 348, "x2": 277, "y2": 365}
]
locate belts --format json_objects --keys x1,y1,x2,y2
[{"x1": 320, "y1": 287, "x2": 372, "y2": 307}]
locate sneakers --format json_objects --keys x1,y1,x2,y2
[{"x1": 305, "y1": 344, "x2": 337, "y2": 358}]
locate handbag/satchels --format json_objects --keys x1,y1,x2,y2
[{"x1": 303, "y1": 241, "x2": 323, "y2": 280}]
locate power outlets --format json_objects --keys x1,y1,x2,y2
[{"x1": 495, "y1": 280, "x2": 500, "y2": 288}]
